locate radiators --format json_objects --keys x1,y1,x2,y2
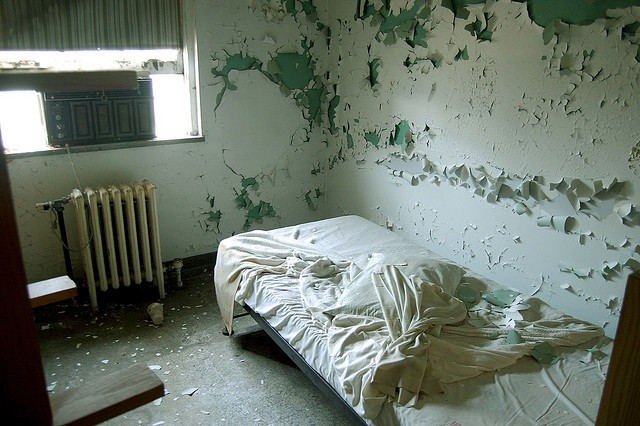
[{"x1": 36, "y1": 179, "x2": 183, "y2": 310}]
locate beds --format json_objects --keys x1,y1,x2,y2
[{"x1": 221, "y1": 215, "x2": 640, "y2": 425}]
